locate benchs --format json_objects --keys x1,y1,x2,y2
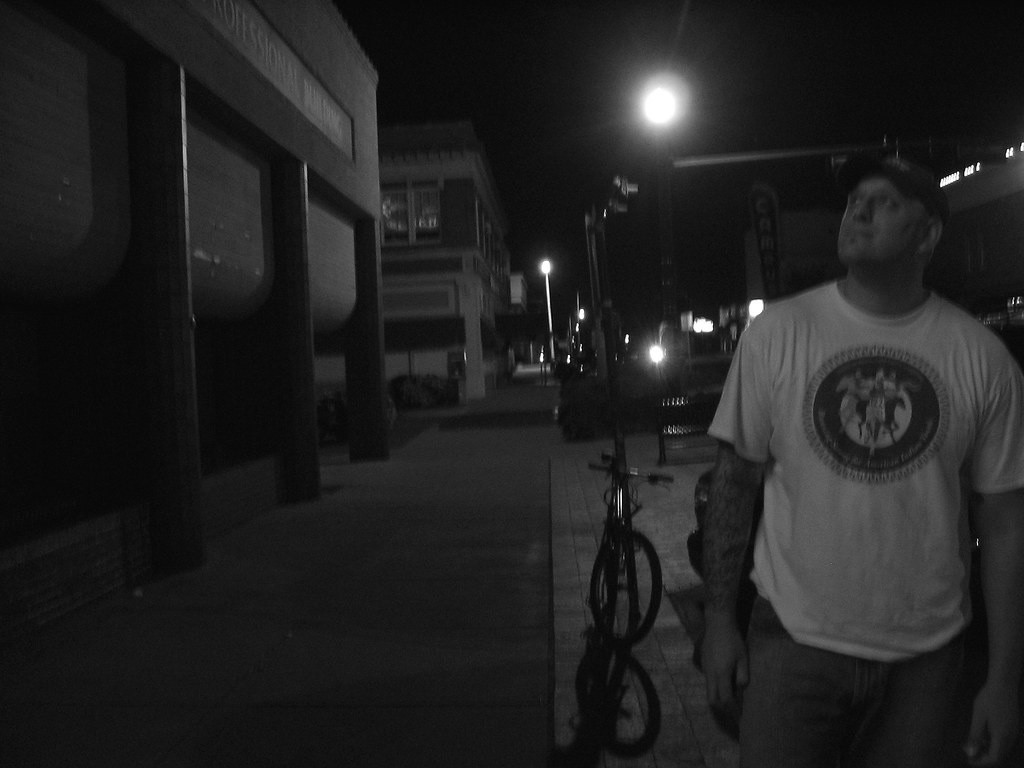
[{"x1": 652, "y1": 391, "x2": 721, "y2": 467}]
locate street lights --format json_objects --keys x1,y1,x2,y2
[
  {"x1": 541, "y1": 259, "x2": 557, "y2": 373},
  {"x1": 625, "y1": 68, "x2": 701, "y2": 396}
]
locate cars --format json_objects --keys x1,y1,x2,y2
[{"x1": 684, "y1": 465, "x2": 764, "y2": 603}]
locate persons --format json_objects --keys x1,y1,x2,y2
[{"x1": 700, "y1": 159, "x2": 1024, "y2": 768}]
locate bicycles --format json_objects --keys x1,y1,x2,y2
[{"x1": 588, "y1": 450, "x2": 676, "y2": 647}]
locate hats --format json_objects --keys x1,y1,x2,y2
[{"x1": 839, "y1": 157, "x2": 949, "y2": 227}]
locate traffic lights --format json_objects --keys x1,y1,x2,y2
[
  {"x1": 612, "y1": 174, "x2": 640, "y2": 200},
  {"x1": 606, "y1": 195, "x2": 630, "y2": 216}
]
